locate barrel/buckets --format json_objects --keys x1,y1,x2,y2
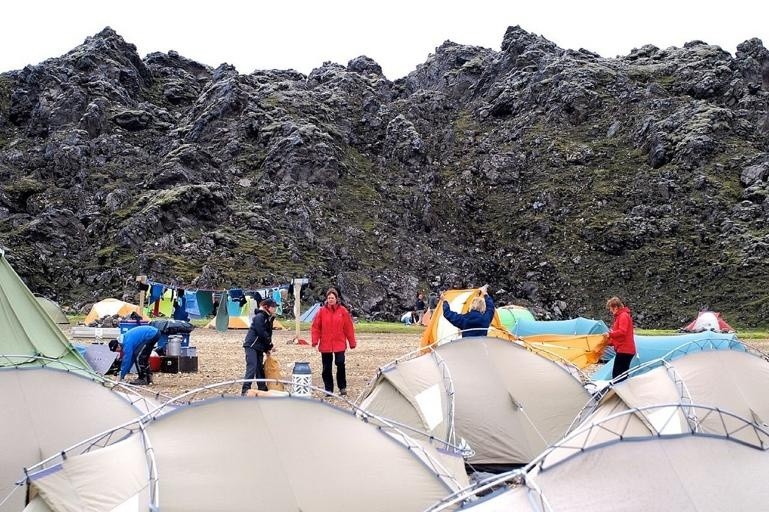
[
  {"x1": 148, "y1": 332, "x2": 199, "y2": 373},
  {"x1": 120, "y1": 321, "x2": 148, "y2": 334}
]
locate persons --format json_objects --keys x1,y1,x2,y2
[
  {"x1": 311, "y1": 289, "x2": 358, "y2": 397},
  {"x1": 401, "y1": 290, "x2": 437, "y2": 328},
  {"x1": 436, "y1": 287, "x2": 496, "y2": 336},
  {"x1": 108, "y1": 326, "x2": 159, "y2": 384},
  {"x1": 602, "y1": 297, "x2": 636, "y2": 379},
  {"x1": 242, "y1": 298, "x2": 283, "y2": 391}
]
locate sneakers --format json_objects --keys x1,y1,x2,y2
[
  {"x1": 130, "y1": 378, "x2": 153, "y2": 385},
  {"x1": 326, "y1": 389, "x2": 347, "y2": 397}
]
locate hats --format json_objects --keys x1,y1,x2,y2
[
  {"x1": 263, "y1": 298, "x2": 280, "y2": 307},
  {"x1": 109, "y1": 340, "x2": 118, "y2": 352}
]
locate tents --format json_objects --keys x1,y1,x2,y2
[
  {"x1": 0, "y1": 332, "x2": 769, "y2": 512},
  {"x1": 685, "y1": 309, "x2": 736, "y2": 334},
  {"x1": 496, "y1": 305, "x2": 536, "y2": 332},
  {"x1": 202, "y1": 298, "x2": 289, "y2": 331},
  {"x1": 299, "y1": 301, "x2": 323, "y2": 323},
  {"x1": 512, "y1": 317, "x2": 609, "y2": 335},
  {"x1": 0, "y1": 244, "x2": 98, "y2": 381},
  {"x1": 84, "y1": 297, "x2": 149, "y2": 326}
]
